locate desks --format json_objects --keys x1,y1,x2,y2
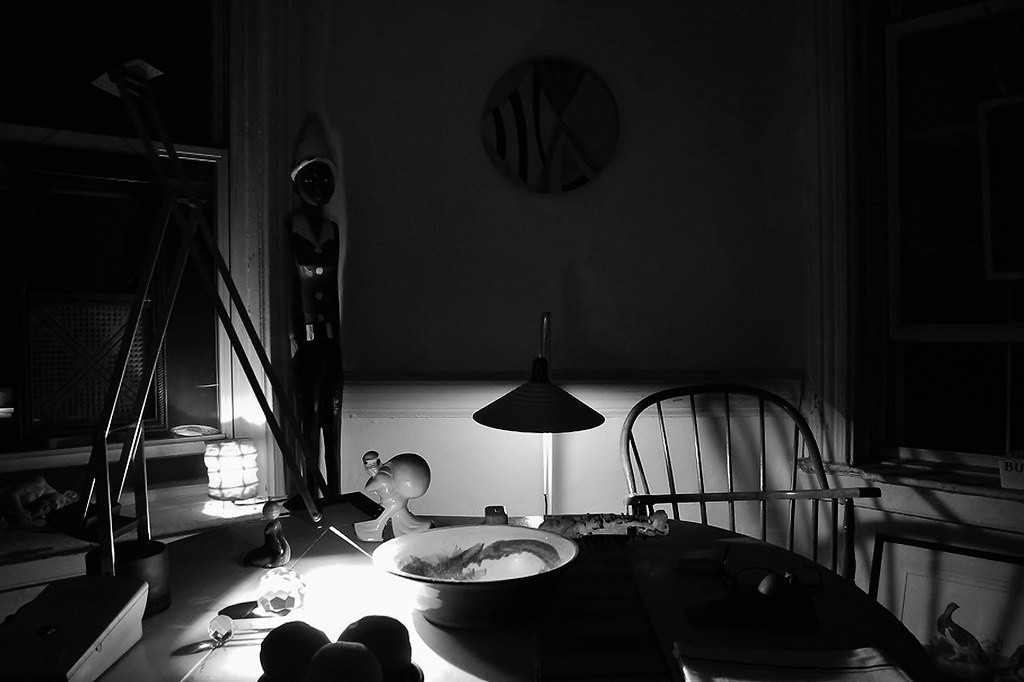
[{"x1": 98, "y1": 512, "x2": 944, "y2": 682}]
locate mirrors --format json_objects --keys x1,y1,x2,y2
[{"x1": 481, "y1": 55, "x2": 620, "y2": 195}]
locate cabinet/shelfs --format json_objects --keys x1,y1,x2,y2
[{"x1": 883, "y1": 0, "x2": 1024, "y2": 470}]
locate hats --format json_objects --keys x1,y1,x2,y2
[{"x1": 290, "y1": 155, "x2": 339, "y2": 182}]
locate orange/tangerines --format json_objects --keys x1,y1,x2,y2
[{"x1": 260, "y1": 616, "x2": 413, "y2": 682}]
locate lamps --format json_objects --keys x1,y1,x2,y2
[{"x1": 471, "y1": 311, "x2": 605, "y2": 527}]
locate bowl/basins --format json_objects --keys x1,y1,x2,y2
[
  {"x1": 257, "y1": 659, "x2": 424, "y2": 682},
  {"x1": 372, "y1": 527, "x2": 579, "y2": 627}
]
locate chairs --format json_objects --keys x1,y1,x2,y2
[{"x1": 625, "y1": 385, "x2": 882, "y2": 582}]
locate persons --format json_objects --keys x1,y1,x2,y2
[{"x1": 284, "y1": 154, "x2": 349, "y2": 512}]
